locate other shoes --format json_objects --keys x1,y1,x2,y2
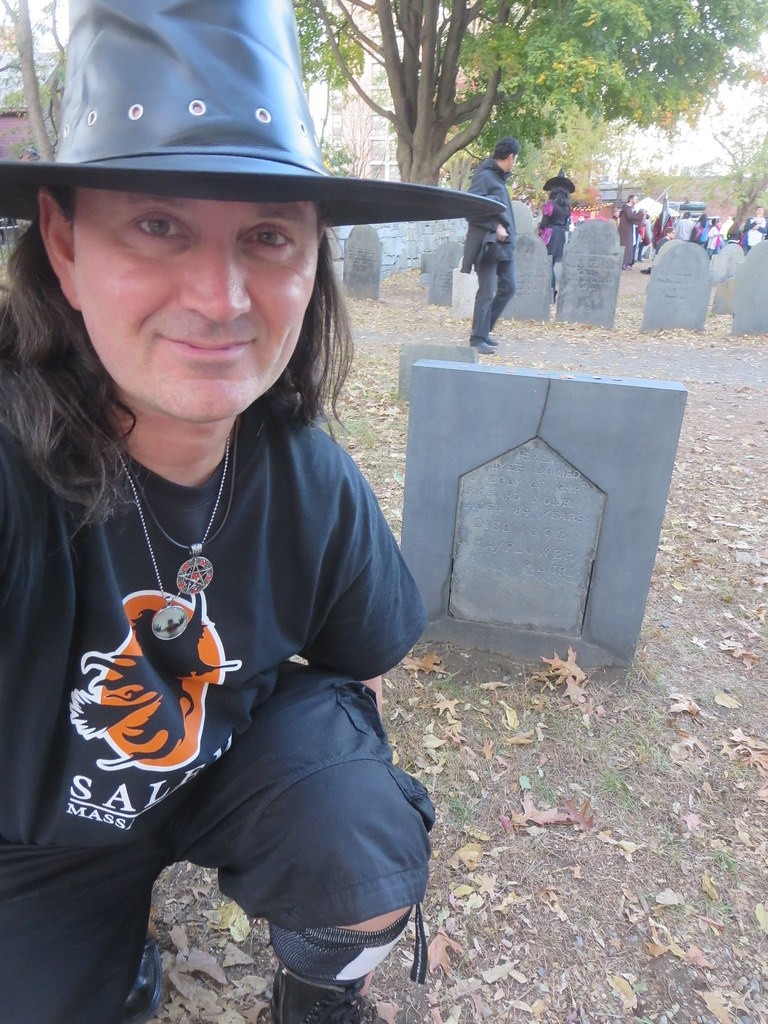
[
  {"x1": 485, "y1": 336, "x2": 499, "y2": 346},
  {"x1": 622, "y1": 264, "x2": 632, "y2": 271},
  {"x1": 471, "y1": 342, "x2": 495, "y2": 354}
]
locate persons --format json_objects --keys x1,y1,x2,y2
[
  {"x1": 0, "y1": 186, "x2": 437, "y2": 1024},
  {"x1": 536, "y1": 186, "x2": 570, "y2": 302},
  {"x1": 613, "y1": 195, "x2": 768, "y2": 274},
  {"x1": 461, "y1": 138, "x2": 522, "y2": 354}
]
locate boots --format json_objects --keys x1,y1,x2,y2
[
  {"x1": 117, "y1": 934, "x2": 163, "y2": 1024},
  {"x1": 269, "y1": 960, "x2": 387, "y2": 1024}
]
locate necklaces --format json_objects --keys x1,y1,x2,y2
[{"x1": 112, "y1": 421, "x2": 238, "y2": 640}]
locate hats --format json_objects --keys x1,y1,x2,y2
[
  {"x1": 543, "y1": 168, "x2": 575, "y2": 193},
  {"x1": 1, "y1": 0, "x2": 508, "y2": 226}
]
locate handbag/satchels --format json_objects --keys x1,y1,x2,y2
[
  {"x1": 696, "y1": 232, "x2": 707, "y2": 243},
  {"x1": 639, "y1": 226, "x2": 646, "y2": 240}
]
